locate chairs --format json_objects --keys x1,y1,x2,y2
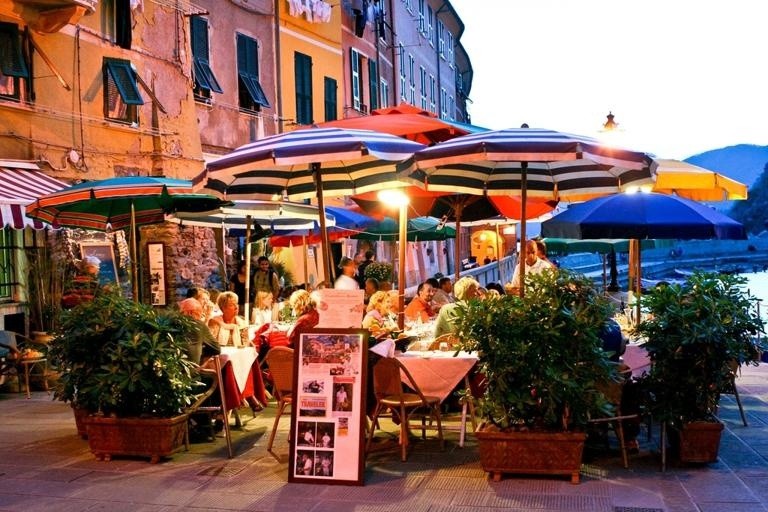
[
  {"x1": 706, "y1": 355, "x2": 747, "y2": 429},
  {"x1": 0, "y1": 330, "x2": 51, "y2": 399}
]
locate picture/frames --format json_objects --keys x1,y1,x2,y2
[
  {"x1": 79, "y1": 242, "x2": 122, "y2": 298},
  {"x1": 287, "y1": 328, "x2": 366, "y2": 486},
  {"x1": 145, "y1": 241, "x2": 168, "y2": 307}
]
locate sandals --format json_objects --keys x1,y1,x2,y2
[{"x1": 246, "y1": 396, "x2": 264, "y2": 412}]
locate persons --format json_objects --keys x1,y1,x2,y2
[
  {"x1": 80, "y1": 255, "x2": 101, "y2": 276},
  {"x1": 180, "y1": 239, "x2": 557, "y2": 412},
  {"x1": 296, "y1": 383, "x2": 347, "y2": 478},
  {"x1": 568, "y1": 278, "x2": 640, "y2": 455}
]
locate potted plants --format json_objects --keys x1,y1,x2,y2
[
  {"x1": 629, "y1": 269, "x2": 768, "y2": 467},
  {"x1": 45, "y1": 284, "x2": 205, "y2": 462},
  {"x1": 444, "y1": 268, "x2": 617, "y2": 484}
]
